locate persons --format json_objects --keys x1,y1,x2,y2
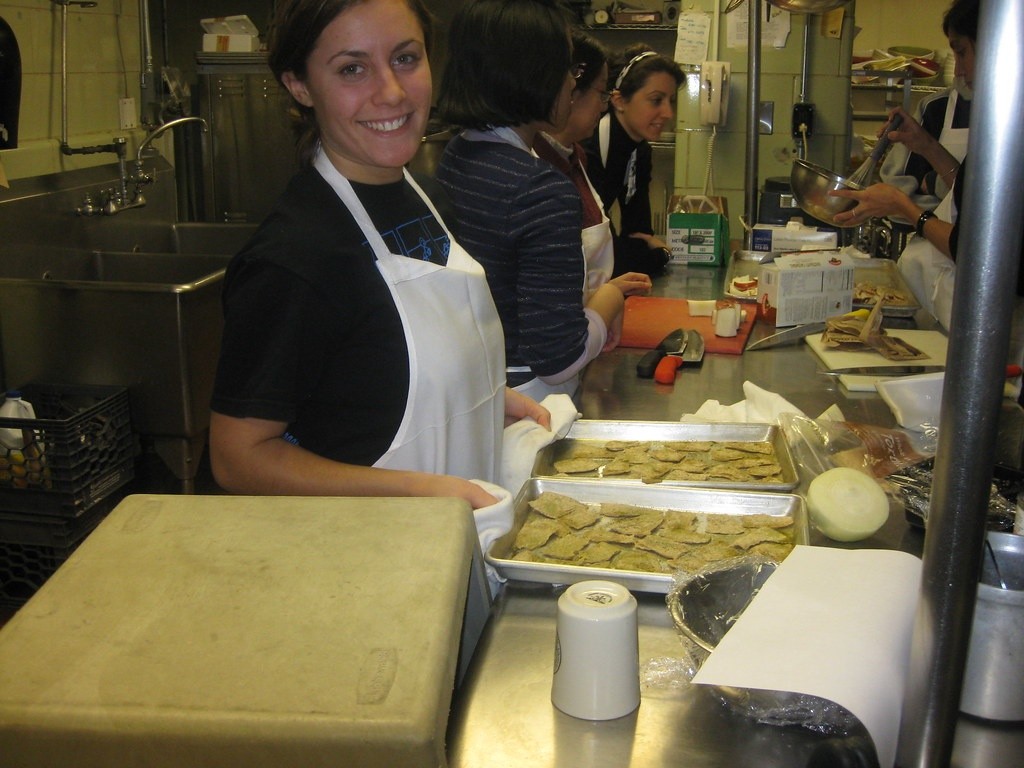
[
  {"x1": 209, "y1": 0, "x2": 553, "y2": 510},
  {"x1": 434, "y1": 0, "x2": 686, "y2": 414},
  {"x1": 827, "y1": 0, "x2": 983, "y2": 332}
]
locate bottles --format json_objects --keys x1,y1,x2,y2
[
  {"x1": 960, "y1": 530, "x2": 1024, "y2": 722},
  {"x1": 0, "y1": 389, "x2": 44, "y2": 454}
]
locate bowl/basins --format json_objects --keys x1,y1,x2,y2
[
  {"x1": 943, "y1": 51, "x2": 956, "y2": 86},
  {"x1": 790, "y1": 158, "x2": 876, "y2": 227},
  {"x1": 669, "y1": 558, "x2": 863, "y2": 722}
]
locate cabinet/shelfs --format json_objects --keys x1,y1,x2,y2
[
  {"x1": 850, "y1": 70, "x2": 914, "y2": 171},
  {"x1": 577, "y1": 22, "x2": 678, "y2": 147}
]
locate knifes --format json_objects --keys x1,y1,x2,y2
[
  {"x1": 638, "y1": 327, "x2": 689, "y2": 376},
  {"x1": 745, "y1": 307, "x2": 872, "y2": 351},
  {"x1": 655, "y1": 327, "x2": 706, "y2": 383},
  {"x1": 820, "y1": 364, "x2": 1020, "y2": 376}
]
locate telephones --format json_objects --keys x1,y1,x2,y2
[{"x1": 700, "y1": 61, "x2": 731, "y2": 128}]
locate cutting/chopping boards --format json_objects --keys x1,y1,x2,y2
[
  {"x1": 796, "y1": 328, "x2": 1022, "y2": 397},
  {"x1": 616, "y1": 295, "x2": 758, "y2": 354}
]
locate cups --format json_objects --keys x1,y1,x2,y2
[{"x1": 551, "y1": 579, "x2": 641, "y2": 720}]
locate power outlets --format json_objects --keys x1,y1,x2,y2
[{"x1": 792, "y1": 102, "x2": 815, "y2": 138}]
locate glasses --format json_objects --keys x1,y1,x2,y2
[
  {"x1": 590, "y1": 86, "x2": 613, "y2": 103},
  {"x1": 567, "y1": 61, "x2": 587, "y2": 78}
]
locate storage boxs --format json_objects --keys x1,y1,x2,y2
[
  {"x1": 0, "y1": 383, "x2": 138, "y2": 619},
  {"x1": 663, "y1": 193, "x2": 731, "y2": 266},
  {"x1": 753, "y1": 221, "x2": 839, "y2": 250},
  {"x1": 199, "y1": 14, "x2": 259, "y2": 53},
  {"x1": 756, "y1": 250, "x2": 855, "y2": 327}
]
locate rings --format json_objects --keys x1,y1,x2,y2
[{"x1": 852, "y1": 209, "x2": 856, "y2": 218}]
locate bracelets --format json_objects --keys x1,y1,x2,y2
[
  {"x1": 940, "y1": 163, "x2": 960, "y2": 178},
  {"x1": 916, "y1": 209, "x2": 938, "y2": 239},
  {"x1": 663, "y1": 247, "x2": 671, "y2": 263}
]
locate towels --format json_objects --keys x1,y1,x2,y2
[
  {"x1": 470, "y1": 394, "x2": 585, "y2": 602},
  {"x1": 682, "y1": 376, "x2": 812, "y2": 454}
]
